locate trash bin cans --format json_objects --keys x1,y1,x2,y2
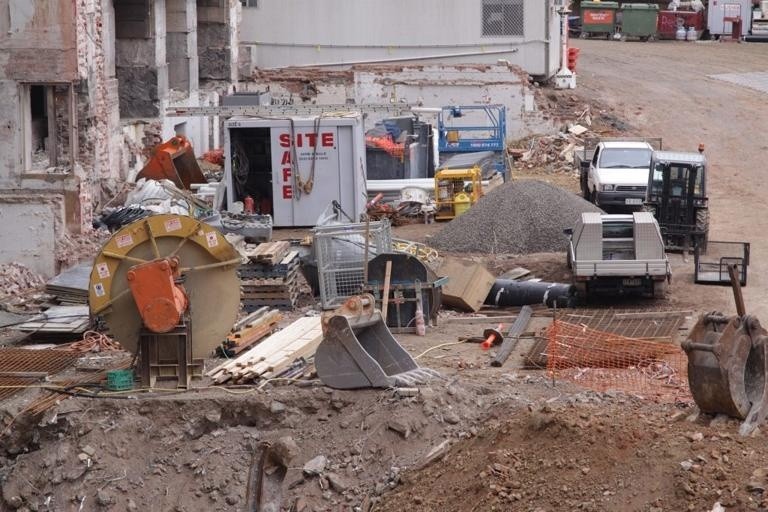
[{"x1": 580, "y1": 0, "x2": 660, "y2": 42}]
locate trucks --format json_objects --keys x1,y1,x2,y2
[
  {"x1": 562, "y1": 210, "x2": 671, "y2": 303},
  {"x1": 571, "y1": 131, "x2": 662, "y2": 211}
]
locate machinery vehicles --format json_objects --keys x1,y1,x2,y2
[{"x1": 642, "y1": 142, "x2": 709, "y2": 263}]
